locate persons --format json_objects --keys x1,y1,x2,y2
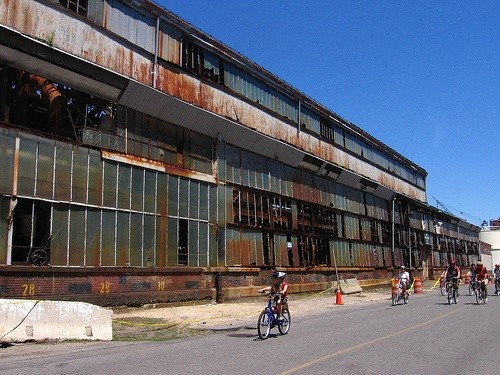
[
  {"x1": 466, "y1": 263, "x2": 476, "y2": 291},
  {"x1": 474, "y1": 261, "x2": 488, "y2": 298},
  {"x1": 257, "y1": 272, "x2": 289, "y2": 320},
  {"x1": 494, "y1": 264, "x2": 500, "y2": 293},
  {"x1": 444, "y1": 260, "x2": 460, "y2": 299},
  {"x1": 391, "y1": 265, "x2": 409, "y2": 295}
]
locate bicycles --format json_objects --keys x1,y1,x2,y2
[
  {"x1": 465, "y1": 273, "x2": 500, "y2": 305},
  {"x1": 444, "y1": 276, "x2": 460, "y2": 305},
  {"x1": 389, "y1": 276, "x2": 410, "y2": 306},
  {"x1": 257, "y1": 290, "x2": 291, "y2": 340}
]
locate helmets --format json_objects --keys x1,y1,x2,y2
[
  {"x1": 274, "y1": 271, "x2": 286, "y2": 279},
  {"x1": 477, "y1": 262, "x2": 483, "y2": 266},
  {"x1": 495, "y1": 264, "x2": 500, "y2": 267},
  {"x1": 448, "y1": 259, "x2": 456, "y2": 264}
]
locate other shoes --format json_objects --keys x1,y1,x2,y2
[{"x1": 277, "y1": 316, "x2": 284, "y2": 321}]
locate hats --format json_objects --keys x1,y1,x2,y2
[{"x1": 400, "y1": 266, "x2": 406, "y2": 269}]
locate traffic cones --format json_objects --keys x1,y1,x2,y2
[{"x1": 333, "y1": 282, "x2": 344, "y2": 305}]
